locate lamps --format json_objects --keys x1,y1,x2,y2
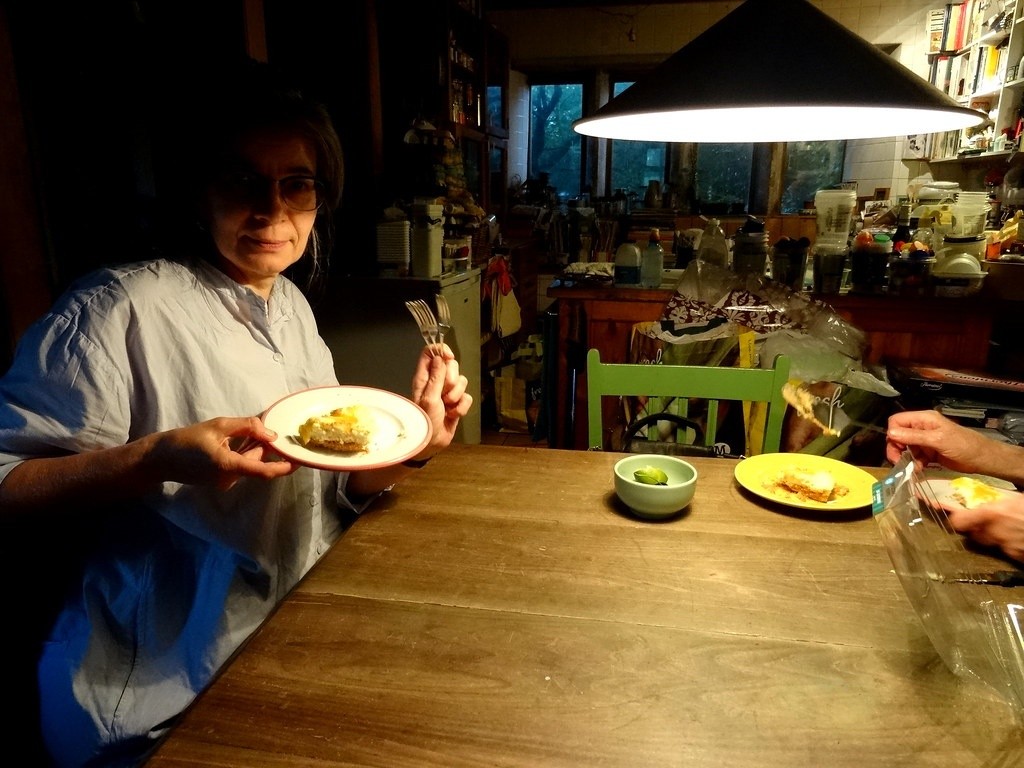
[{"x1": 571, "y1": 0, "x2": 988, "y2": 143}]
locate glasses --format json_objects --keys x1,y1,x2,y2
[{"x1": 218, "y1": 172, "x2": 330, "y2": 211}]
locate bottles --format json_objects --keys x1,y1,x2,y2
[
  {"x1": 892, "y1": 206, "x2": 911, "y2": 244},
  {"x1": 853, "y1": 228, "x2": 874, "y2": 249},
  {"x1": 911, "y1": 207, "x2": 933, "y2": 249},
  {"x1": 614, "y1": 238, "x2": 641, "y2": 288},
  {"x1": 873, "y1": 234, "x2": 893, "y2": 253},
  {"x1": 642, "y1": 228, "x2": 663, "y2": 288},
  {"x1": 699, "y1": 219, "x2": 728, "y2": 270},
  {"x1": 932, "y1": 205, "x2": 953, "y2": 252}
]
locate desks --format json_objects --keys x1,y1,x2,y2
[{"x1": 147, "y1": 441, "x2": 1024, "y2": 768}]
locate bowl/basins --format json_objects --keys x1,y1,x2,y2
[
  {"x1": 614, "y1": 454, "x2": 697, "y2": 519},
  {"x1": 937, "y1": 254, "x2": 981, "y2": 272}
]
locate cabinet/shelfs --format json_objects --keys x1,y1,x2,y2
[
  {"x1": 926, "y1": 0, "x2": 1024, "y2": 167},
  {"x1": 546, "y1": 275, "x2": 995, "y2": 450},
  {"x1": 385, "y1": 1, "x2": 509, "y2": 241}
]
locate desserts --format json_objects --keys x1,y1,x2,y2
[{"x1": 633, "y1": 468, "x2": 669, "y2": 486}]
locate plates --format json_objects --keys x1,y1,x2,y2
[
  {"x1": 734, "y1": 453, "x2": 878, "y2": 511},
  {"x1": 261, "y1": 385, "x2": 432, "y2": 472},
  {"x1": 910, "y1": 477, "x2": 1023, "y2": 512}
]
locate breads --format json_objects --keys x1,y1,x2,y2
[
  {"x1": 778, "y1": 467, "x2": 835, "y2": 503},
  {"x1": 298, "y1": 402, "x2": 373, "y2": 453},
  {"x1": 951, "y1": 476, "x2": 1003, "y2": 510}
]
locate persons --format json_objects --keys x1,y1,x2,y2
[
  {"x1": 0, "y1": 88, "x2": 474, "y2": 768},
  {"x1": 886, "y1": 410, "x2": 1024, "y2": 568}
]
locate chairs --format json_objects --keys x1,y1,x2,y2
[{"x1": 586, "y1": 348, "x2": 788, "y2": 458}]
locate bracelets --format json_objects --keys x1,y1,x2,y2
[{"x1": 402, "y1": 457, "x2": 433, "y2": 469}]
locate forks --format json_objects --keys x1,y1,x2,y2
[{"x1": 405, "y1": 295, "x2": 452, "y2": 358}]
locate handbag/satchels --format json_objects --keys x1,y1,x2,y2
[
  {"x1": 493, "y1": 333, "x2": 551, "y2": 441},
  {"x1": 34, "y1": 501, "x2": 274, "y2": 759},
  {"x1": 621, "y1": 315, "x2": 750, "y2": 458},
  {"x1": 618, "y1": 413, "x2": 714, "y2": 456}
]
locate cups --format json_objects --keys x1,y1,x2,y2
[
  {"x1": 851, "y1": 247, "x2": 888, "y2": 297},
  {"x1": 814, "y1": 189, "x2": 857, "y2": 249},
  {"x1": 733, "y1": 227, "x2": 769, "y2": 276},
  {"x1": 811, "y1": 245, "x2": 848, "y2": 293},
  {"x1": 769, "y1": 245, "x2": 810, "y2": 292},
  {"x1": 955, "y1": 191, "x2": 989, "y2": 212}
]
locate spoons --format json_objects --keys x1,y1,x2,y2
[{"x1": 813, "y1": 404, "x2": 888, "y2": 434}]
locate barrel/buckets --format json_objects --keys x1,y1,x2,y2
[{"x1": 943, "y1": 234, "x2": 987, "y2": 263}]
